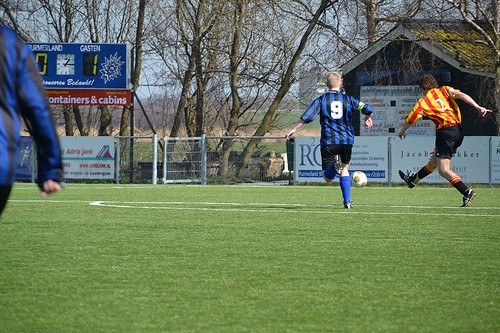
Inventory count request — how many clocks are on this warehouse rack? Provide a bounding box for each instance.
[56,52,75,76]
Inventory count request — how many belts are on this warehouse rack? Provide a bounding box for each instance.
[440,126,458,131]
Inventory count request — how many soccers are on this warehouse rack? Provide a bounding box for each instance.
[351,170,368,187]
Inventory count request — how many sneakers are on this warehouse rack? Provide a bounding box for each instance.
[345,203,353,209]
[399,170,417,188]
[460,189,476,207]
[334,155,342,175]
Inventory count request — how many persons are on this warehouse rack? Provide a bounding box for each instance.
[0,22,63,217]
[399,73,492,207]
[285,72,374,208]
